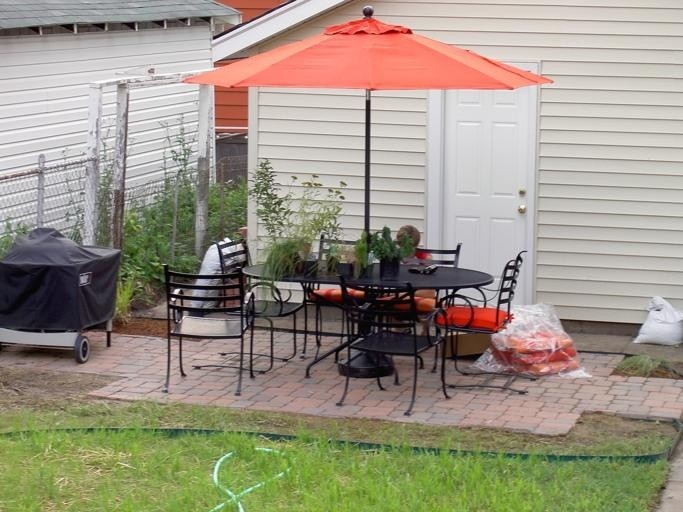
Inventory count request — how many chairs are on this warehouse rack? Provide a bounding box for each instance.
[338,277,450,415]
[163,264,254,394]
[306,232,365,347]
[191,240,304,373]
[433,250,538,394]
[373,243,462,373]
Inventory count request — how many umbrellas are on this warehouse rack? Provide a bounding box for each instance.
[181,6,554,378]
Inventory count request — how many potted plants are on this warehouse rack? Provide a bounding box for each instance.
[249,157,417,308]
